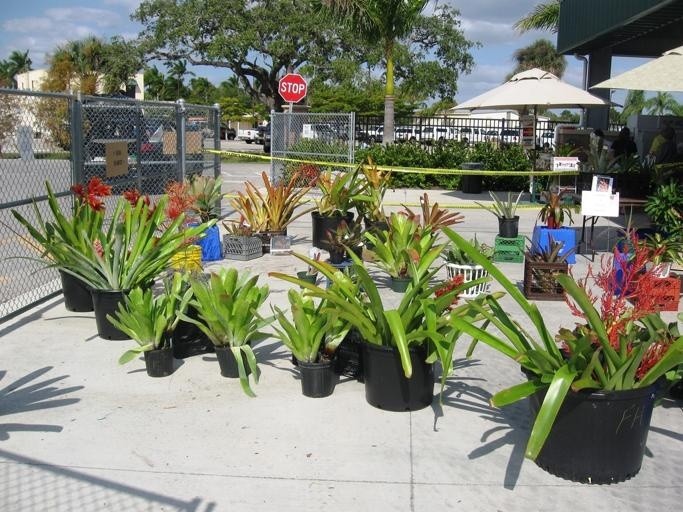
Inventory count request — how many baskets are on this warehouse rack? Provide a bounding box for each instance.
[493,235,525,263]
[445,261,488,299]
[222,234,263,261]
[523,253,568,300]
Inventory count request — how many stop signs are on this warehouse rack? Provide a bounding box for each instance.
[278,73,308,102]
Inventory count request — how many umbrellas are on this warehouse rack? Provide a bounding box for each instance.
[448,67,624,203]
[588,46,683,91]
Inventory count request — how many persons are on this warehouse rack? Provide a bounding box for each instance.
[590,129,613,148]
[649,135,667,155]
[607,126,638,170]
[655,127,678,164]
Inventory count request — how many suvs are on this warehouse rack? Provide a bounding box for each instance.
[194,121,237,140]
[77,94,205,194]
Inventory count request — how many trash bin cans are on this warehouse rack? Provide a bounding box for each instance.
[461,163,483,194]
